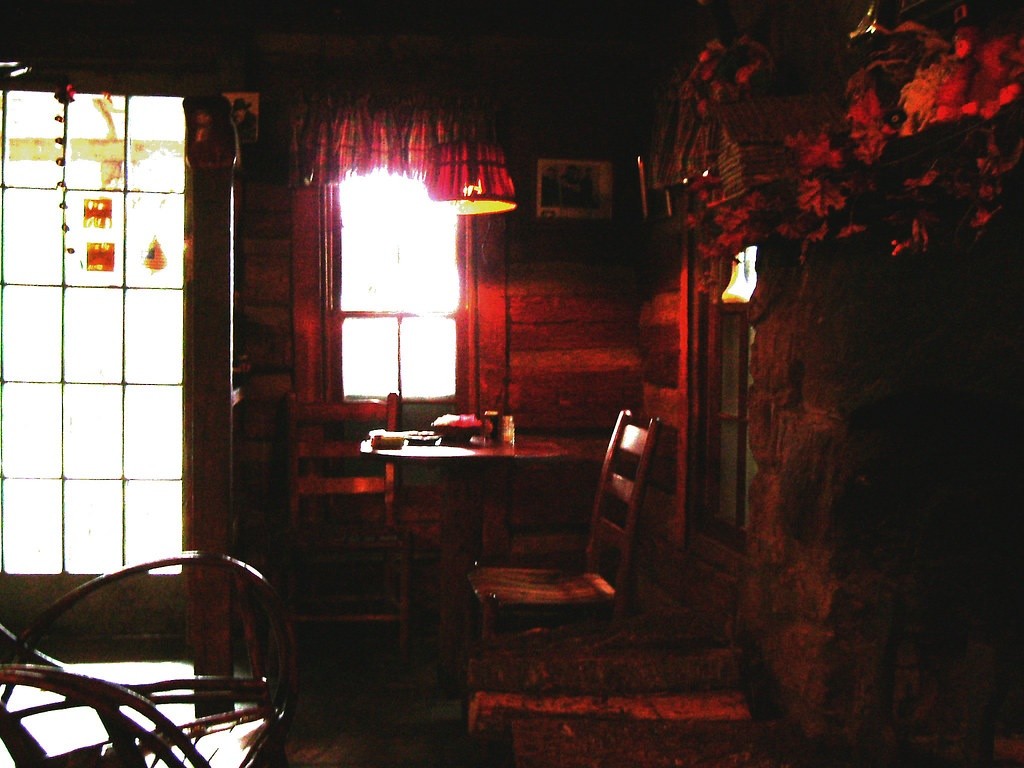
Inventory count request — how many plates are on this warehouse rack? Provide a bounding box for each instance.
[406,436,440,443]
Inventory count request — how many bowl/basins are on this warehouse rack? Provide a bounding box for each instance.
[430,424,481,443]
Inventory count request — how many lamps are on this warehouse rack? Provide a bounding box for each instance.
[416,136,519,216]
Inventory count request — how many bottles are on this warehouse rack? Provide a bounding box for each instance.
[502,415,515,446]
[386,393,400,431]
[483,411,499,443]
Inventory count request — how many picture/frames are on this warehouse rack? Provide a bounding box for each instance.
[527,153,619,222]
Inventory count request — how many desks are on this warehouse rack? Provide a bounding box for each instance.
[360,436,593,637]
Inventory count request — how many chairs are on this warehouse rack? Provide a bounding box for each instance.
[464,407,662,656]
[0,552,298,768]
[269,392,410,670]
